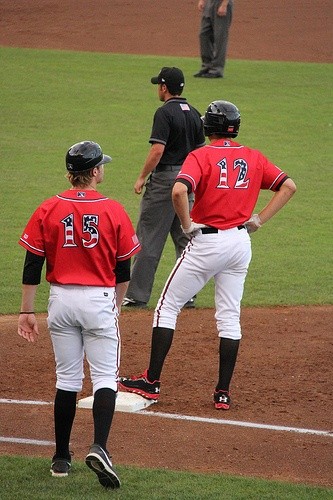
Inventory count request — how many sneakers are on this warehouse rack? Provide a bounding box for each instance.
[121,298,146,307]
[50,451,74,477]
[212,388,231,410]
[184,299,195,308]
[85,444,121,489]
[117,368,161,400]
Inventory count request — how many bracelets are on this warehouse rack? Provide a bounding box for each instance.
[18,311,35,314]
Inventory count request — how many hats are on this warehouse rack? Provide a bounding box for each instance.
[151,67,185,88]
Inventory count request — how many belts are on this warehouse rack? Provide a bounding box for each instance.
[157,165,181,171]
[200,224,245,234]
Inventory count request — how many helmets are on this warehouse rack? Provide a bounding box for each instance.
[66,141,112,172]
[200,100,241,138]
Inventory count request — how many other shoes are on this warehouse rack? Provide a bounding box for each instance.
[193,71,223,78]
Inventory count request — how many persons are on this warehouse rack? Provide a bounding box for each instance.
[119,66,205,308]
[193,0,234,78]
[14,142,141,488]
[120,101,298,408]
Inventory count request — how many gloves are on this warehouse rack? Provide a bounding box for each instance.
[245,214,262,233]
[180,222,206,240]
